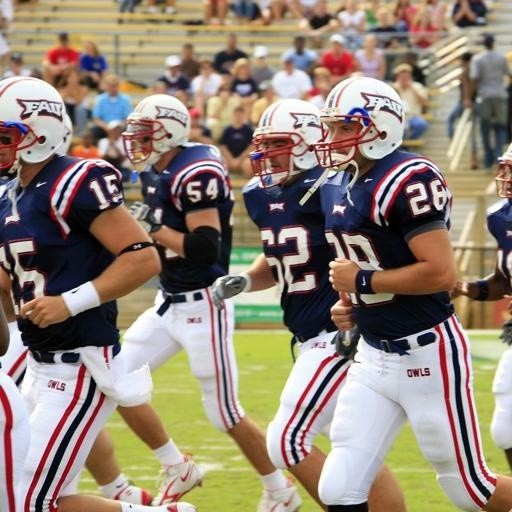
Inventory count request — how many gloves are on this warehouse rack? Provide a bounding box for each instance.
[128,199,162,237]
[210,272,248,310]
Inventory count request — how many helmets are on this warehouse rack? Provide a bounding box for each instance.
[252,98,330,170]
[322,77,405,161]
[127,93,191,154]
[1,75,69,165]
[496,139,512,170]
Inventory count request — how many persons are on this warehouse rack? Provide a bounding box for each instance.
[0,77,198,512]
[308,79,512,511]
[211,97,409,512]
[448,144,512,473]
[0,295,28,512]
[0,113,153,505]
[1,1,512,186]
[111,94,304,512]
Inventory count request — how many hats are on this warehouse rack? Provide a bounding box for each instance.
[253,43,270,59]
[164,55,184,68]
[330,34,345,45]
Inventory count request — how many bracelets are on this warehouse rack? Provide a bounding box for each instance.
[355,266,374,294]
[60,280,101,318]
[476,278,490,303]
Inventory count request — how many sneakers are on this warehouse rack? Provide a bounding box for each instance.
[150,459,204,506]
[254,484,305,512]
[111,483,154,505]
[166,501,197,512]
